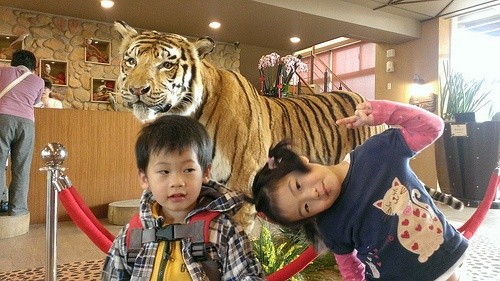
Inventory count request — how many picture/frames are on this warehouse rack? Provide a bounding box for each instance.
[84,38,111,66]
[38,59,69,87]
[0,34,24,62]
[90,77,118,104]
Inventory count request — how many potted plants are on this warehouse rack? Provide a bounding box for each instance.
[440,60,491,124]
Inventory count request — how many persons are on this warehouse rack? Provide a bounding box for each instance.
[101,114,265,281]
[37,78,62,108]
[245,100,470,281]
[0,49,44,217]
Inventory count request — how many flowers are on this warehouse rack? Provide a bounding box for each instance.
[257,52,309,98]
[247,226,314,281]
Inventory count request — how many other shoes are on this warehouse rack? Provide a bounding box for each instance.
[0,200,9,212]
